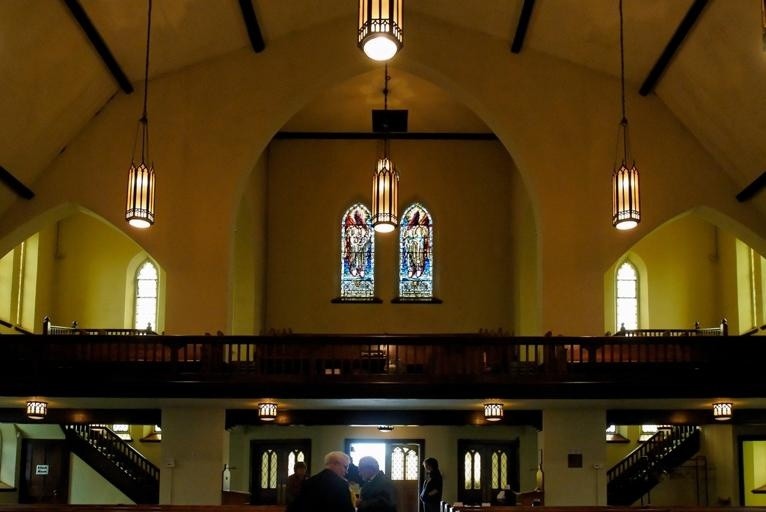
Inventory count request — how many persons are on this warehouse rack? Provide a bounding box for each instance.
[285,461,314,512]
[418,457,443,511]
[356,455,398,512]
[286,451,357,511]
[345,456,361,482]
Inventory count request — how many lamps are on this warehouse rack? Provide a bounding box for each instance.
[610,0,643,231]
[711,400,734,423]
[25,400,49,421]
[370,65,399,235]
[377,424,395,433]
[483,402,506,422]
[356,0,405,64]
[125,0,157,230]
[256,401,278,421]
